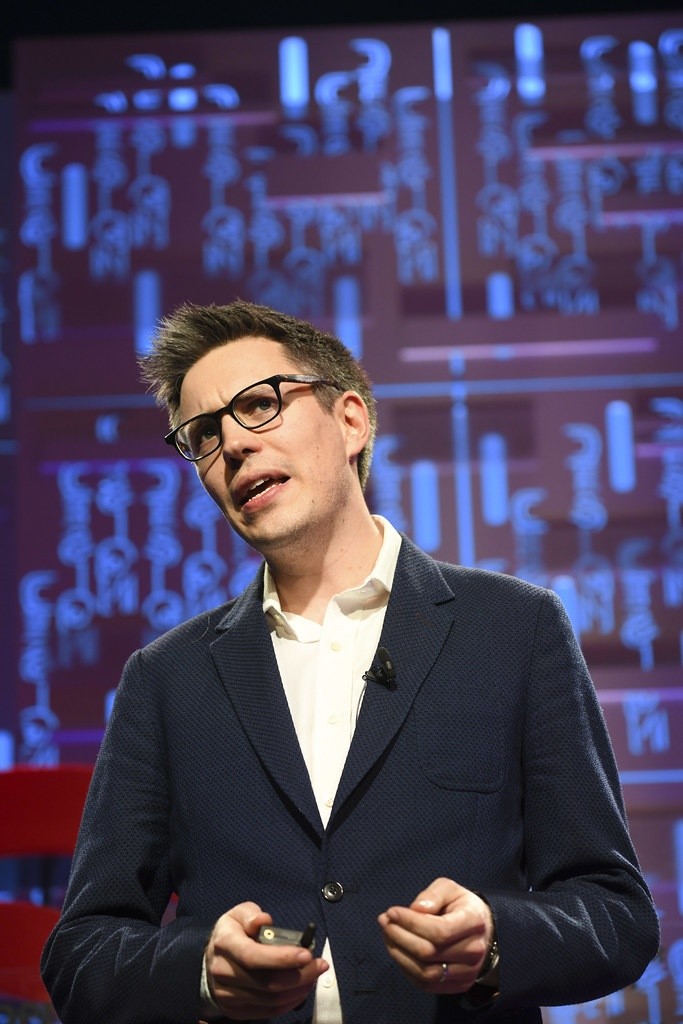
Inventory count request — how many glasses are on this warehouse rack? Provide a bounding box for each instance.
[163,373,346,462]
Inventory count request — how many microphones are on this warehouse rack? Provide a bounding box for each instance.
[362,646,398,689]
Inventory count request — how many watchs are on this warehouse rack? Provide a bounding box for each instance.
[479,917,503,982]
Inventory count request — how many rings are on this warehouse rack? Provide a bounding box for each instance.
[436,960,449,982]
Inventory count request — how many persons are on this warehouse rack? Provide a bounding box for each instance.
[38,305,663,1024]
